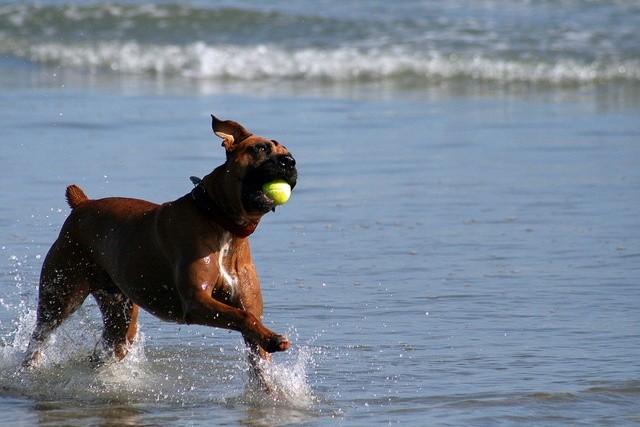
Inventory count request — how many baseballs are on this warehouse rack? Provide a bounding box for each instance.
[262,178,291,205]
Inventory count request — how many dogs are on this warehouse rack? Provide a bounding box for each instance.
[22,114,297,395]
